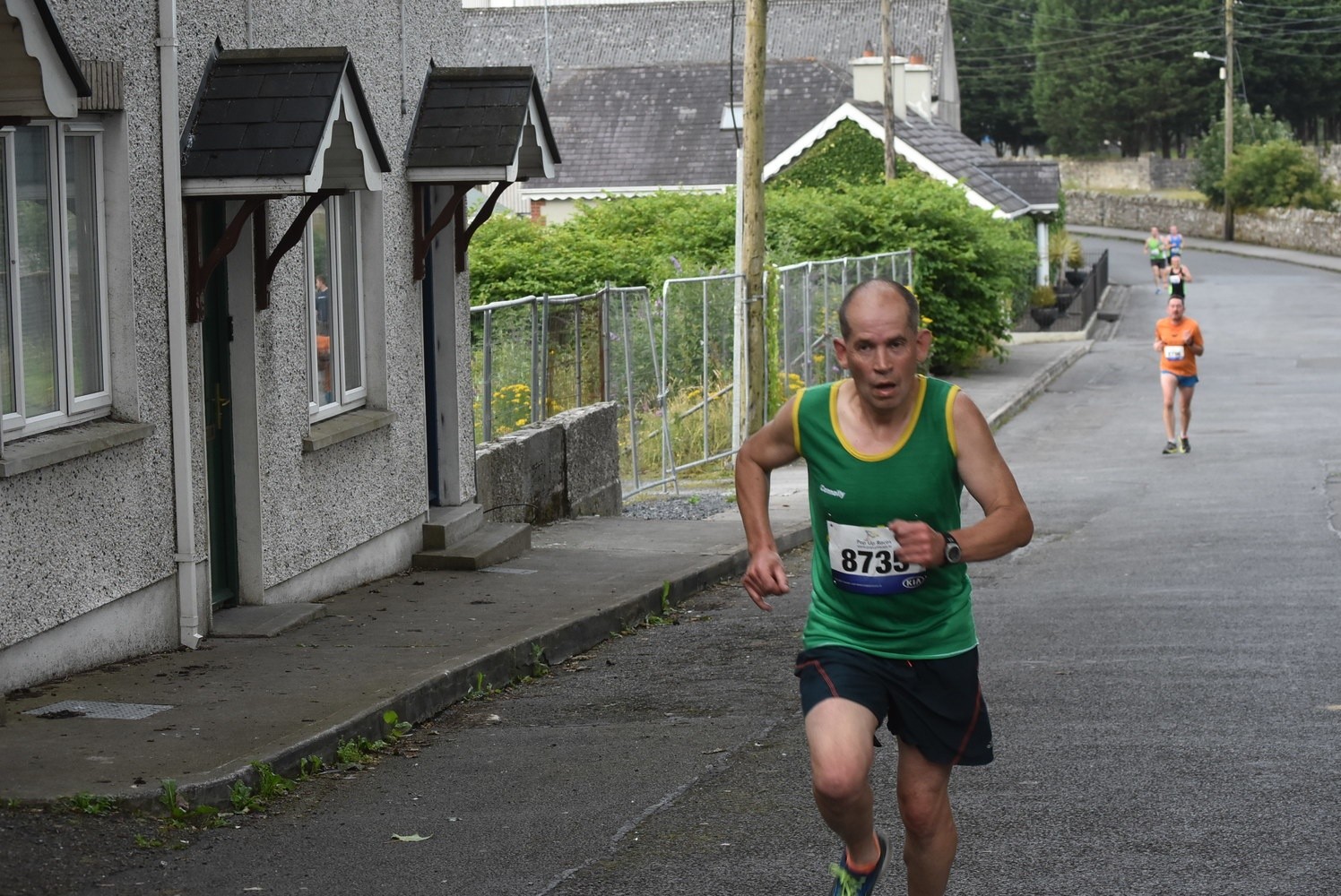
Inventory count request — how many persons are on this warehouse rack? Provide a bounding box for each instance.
[310,273,335,406]
[1144,226,1168,295]
[1166,226,1185,264]
[734,276,1034,896]
[1153,294,1204,454]
[1162,253,1192,300]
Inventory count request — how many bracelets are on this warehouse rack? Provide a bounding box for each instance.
[1184,341,1194,347]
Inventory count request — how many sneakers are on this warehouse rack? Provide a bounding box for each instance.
[831,832,892,896]
[1178,435,1190,453]
[1163,442,1175,453]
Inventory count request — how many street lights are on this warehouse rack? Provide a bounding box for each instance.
[1193,51,1235,242]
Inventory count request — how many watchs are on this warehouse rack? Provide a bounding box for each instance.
[939,530,962,569]
